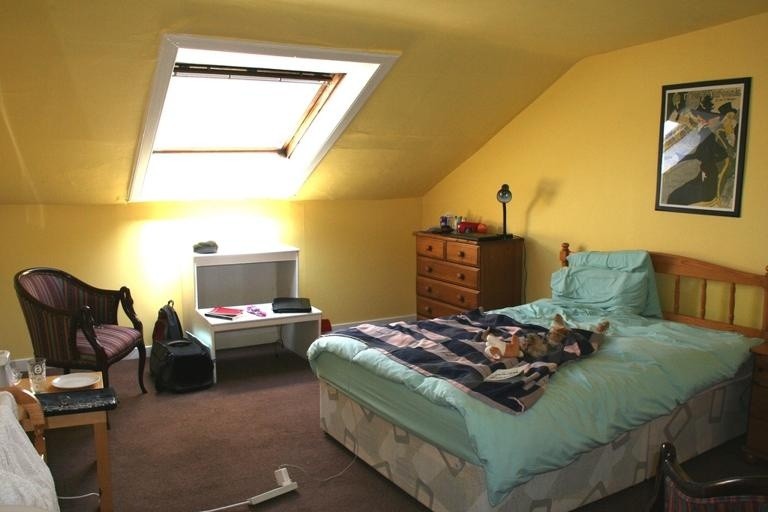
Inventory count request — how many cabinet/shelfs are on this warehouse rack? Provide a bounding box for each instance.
[412,229,524,322]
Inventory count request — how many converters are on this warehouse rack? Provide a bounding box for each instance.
[274,468,291,486]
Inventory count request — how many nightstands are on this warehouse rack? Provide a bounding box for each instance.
[745,340,768,462]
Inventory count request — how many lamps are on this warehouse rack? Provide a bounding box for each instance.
[495,183,513,240]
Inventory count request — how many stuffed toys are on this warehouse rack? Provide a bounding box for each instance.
[484,334,524,363]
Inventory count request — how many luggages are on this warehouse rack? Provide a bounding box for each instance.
[149,338,214,395]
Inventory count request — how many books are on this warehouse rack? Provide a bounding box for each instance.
[205,305,244,320]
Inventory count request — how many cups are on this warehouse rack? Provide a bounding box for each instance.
[25,357,47,394]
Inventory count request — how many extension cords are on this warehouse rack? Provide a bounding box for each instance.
[246,480,299,506]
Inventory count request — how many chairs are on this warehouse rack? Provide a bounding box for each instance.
[14,266,148,400]
[640,440,768,512]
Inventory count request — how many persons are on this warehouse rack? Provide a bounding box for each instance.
[665,103,738,207]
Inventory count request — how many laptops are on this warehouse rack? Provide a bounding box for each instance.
[457,233,500,241]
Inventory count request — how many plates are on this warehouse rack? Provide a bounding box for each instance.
[51,373,100,389]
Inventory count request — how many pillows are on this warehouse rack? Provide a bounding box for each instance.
[551,266,648,318]
[565,251,664,320]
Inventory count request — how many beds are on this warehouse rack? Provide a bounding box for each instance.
[306,242,768,511]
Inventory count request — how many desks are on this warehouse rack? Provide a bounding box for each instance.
[13,373,112,511]
[197,298,321,386]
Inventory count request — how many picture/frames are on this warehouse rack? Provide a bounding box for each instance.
[654,76,753,219]
